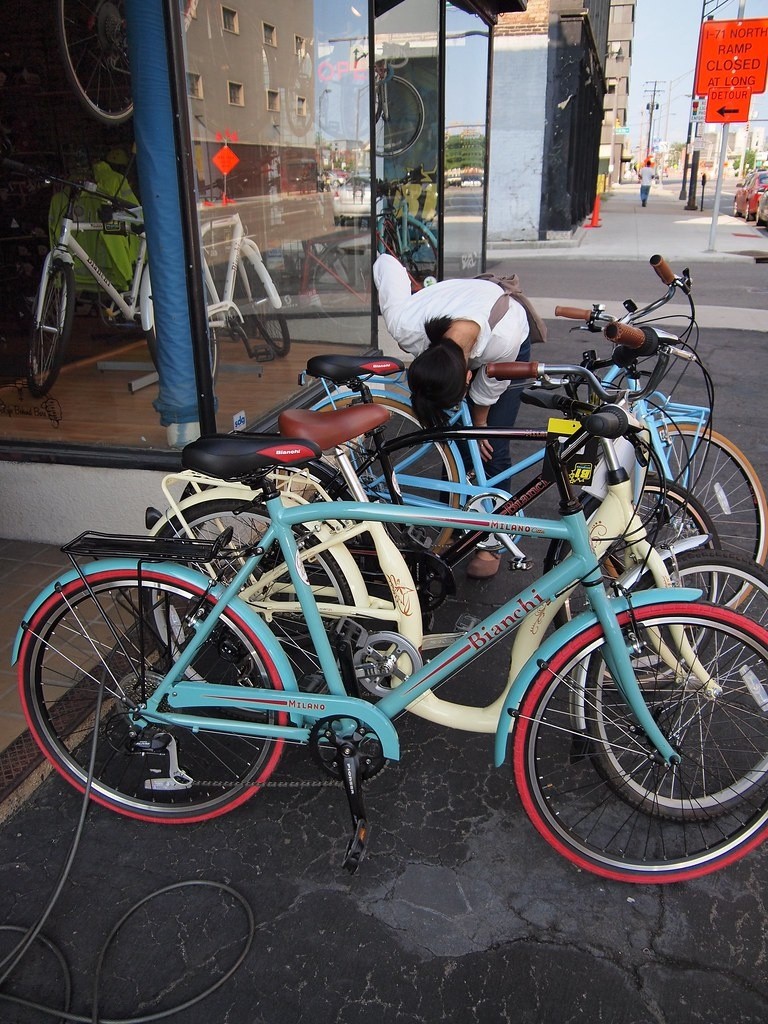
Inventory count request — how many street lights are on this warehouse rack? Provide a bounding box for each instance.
[318,88,333,191]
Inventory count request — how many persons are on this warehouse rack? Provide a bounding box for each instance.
[373,253,530,575]
[48,157,148,293]
[638,161,654,207]
[317,172,330,192]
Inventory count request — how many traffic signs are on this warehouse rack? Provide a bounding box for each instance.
[705,85,752,123]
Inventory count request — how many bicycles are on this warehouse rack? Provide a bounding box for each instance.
[1,153,768,887]
[53,0,133,128]
[375,41,426,159]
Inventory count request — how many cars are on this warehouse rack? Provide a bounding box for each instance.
[755,186,768,227]
[733,170,768,221]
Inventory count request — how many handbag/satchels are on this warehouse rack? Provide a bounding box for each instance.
[481,273,548,344]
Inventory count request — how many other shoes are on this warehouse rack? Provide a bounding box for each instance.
[467,550,502,579]
[441,541,454,554]
